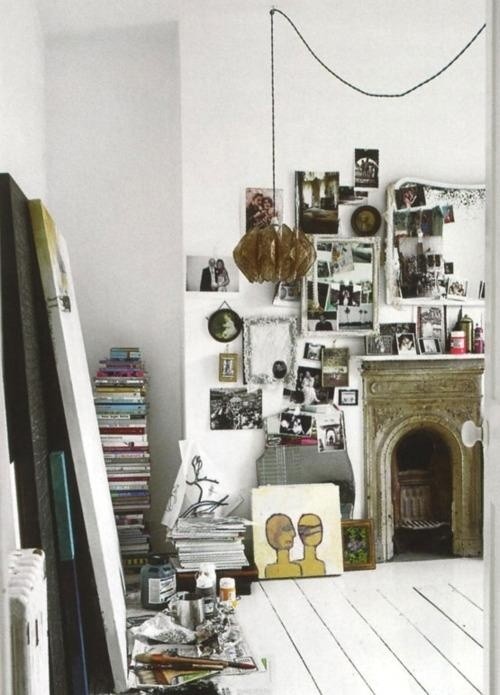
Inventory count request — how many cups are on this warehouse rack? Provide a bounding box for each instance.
[170,592,204,630]
[450,331,466,352]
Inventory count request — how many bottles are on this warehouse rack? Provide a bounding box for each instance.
[219,577,237,610]
[459,314,473,353]
[195,562,217,619]
[474,323,484,353]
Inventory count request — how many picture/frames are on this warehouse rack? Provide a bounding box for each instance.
[339,518,377,572]
[218,353,239,382]
[338,388,359,407]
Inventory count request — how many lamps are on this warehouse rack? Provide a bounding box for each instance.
[232,8,486,288]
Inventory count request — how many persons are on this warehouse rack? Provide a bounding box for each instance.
[407,213,432,237]
[401,188,418,208]
[316,314,333,330]
[198,259,230,291]
[399,335,416,354]
[334,288,357,308]
[283,415,304,434]
[296,371,330,403]
[449,281,465,296]
[246,192,277,232]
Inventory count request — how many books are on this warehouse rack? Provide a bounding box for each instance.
[91,358,262,569]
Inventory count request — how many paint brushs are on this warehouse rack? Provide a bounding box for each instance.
[135,653,256,669]
